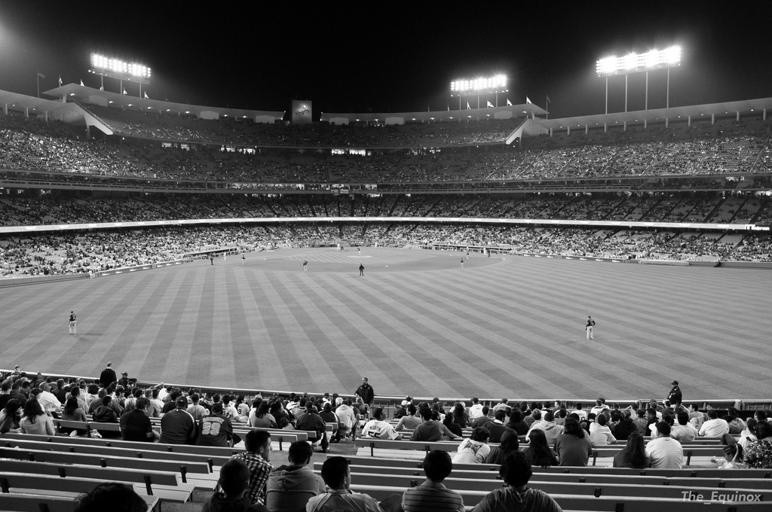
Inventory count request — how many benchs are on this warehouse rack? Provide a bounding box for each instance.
[0,371,770,510]
[211,118,461,251]
[461,114,772,267]
[0,99,210,283]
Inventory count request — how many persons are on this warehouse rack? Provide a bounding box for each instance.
[0,362,770,470]
[471,451,568,511]
[583,315,595,341]
[227,430,273,504]
[305,456,382,511]
[402,449,464,511]
[266,441,324,512]
[200,460,272,511]
[1,104,771,279]
[72,481,148,512]
[67,309,79,337]
